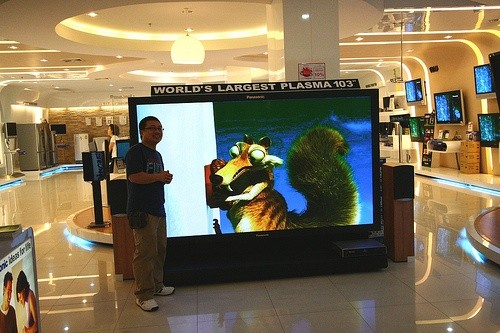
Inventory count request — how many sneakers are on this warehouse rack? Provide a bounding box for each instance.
[153,287,175,295]
[136,298,159,311]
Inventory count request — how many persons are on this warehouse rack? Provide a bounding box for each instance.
[125,116,175,312]
[108,124,120,172]
[0,272,18,333]
[16,270,38,333]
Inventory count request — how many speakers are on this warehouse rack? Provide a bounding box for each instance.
[82,152,105,181]
[108,175,135,280]
[382,164,415,263]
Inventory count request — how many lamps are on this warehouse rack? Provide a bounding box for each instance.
[170,32,205,65]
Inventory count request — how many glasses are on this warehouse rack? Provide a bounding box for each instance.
[142,127,164,131]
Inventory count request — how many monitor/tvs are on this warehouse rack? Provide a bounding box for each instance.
[474,63,495,94]
[390,114,410,128]
[434,89,466,126]
[116,140,130,159]
[478,112,500,148]
[405,78,423,103]
